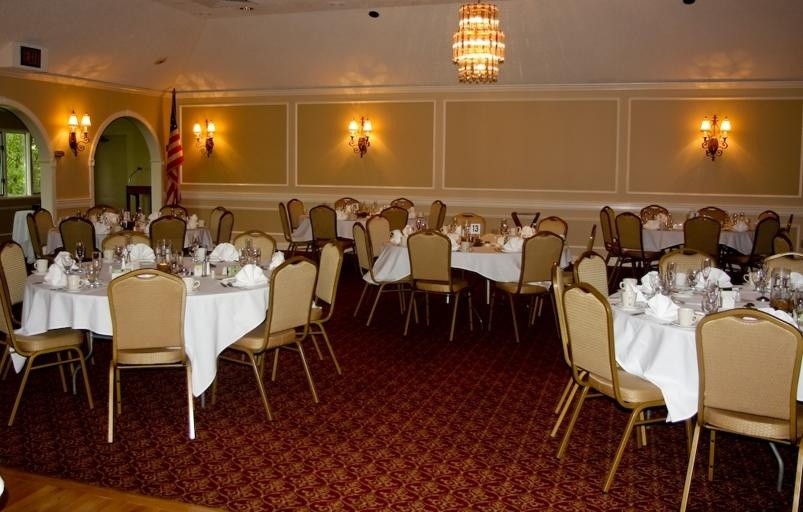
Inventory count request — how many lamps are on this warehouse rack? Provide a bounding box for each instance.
[67,109,92,157]
[348,117,370,158]
[192,120,214,158]
[451,0,505,83]
[700,115,730,161]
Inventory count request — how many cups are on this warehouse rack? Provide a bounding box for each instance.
[393,211,538,253]
[29,236,261,295]
[608,258,803,336]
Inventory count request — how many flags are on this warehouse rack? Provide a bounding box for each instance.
[164,93,185,207]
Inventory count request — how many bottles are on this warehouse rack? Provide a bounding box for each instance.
[301,198,391,221]
[56,205,206,235]
[641,208,759,232]
